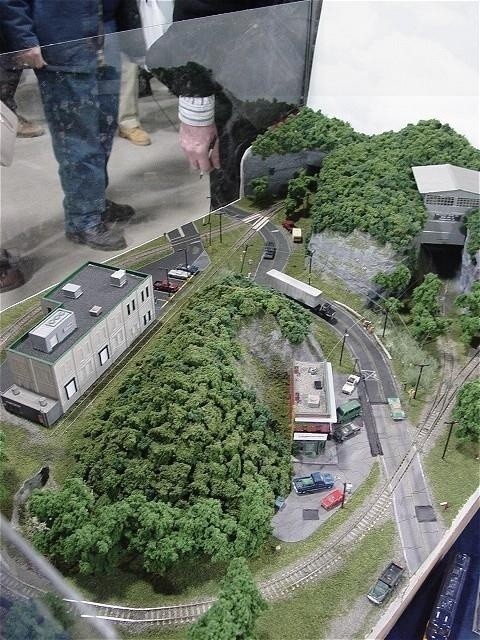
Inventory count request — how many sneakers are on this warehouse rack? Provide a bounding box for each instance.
[64,220,128,251]
[99,197,134,221]
[118,122,152,146]
[9,110,46,138]
[0,252,25,293]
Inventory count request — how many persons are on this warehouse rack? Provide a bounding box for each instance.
[2,1,325,293]
